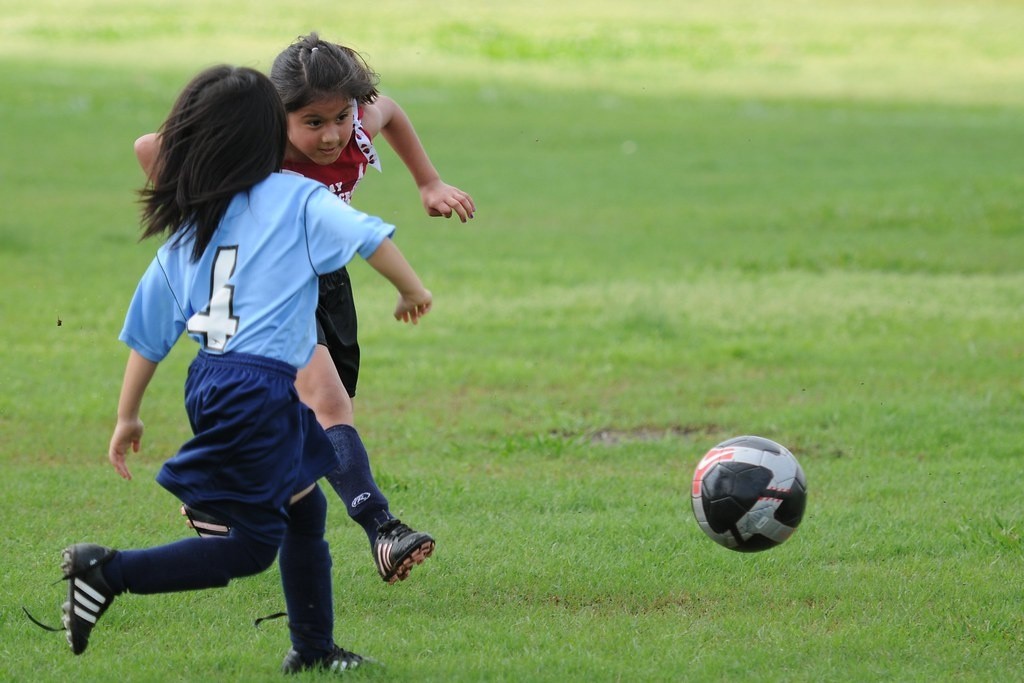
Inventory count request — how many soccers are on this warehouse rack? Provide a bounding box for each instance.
[690,435,808,553]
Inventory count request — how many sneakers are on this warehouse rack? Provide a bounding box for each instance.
[280,646,387,673]
[179,502,229,539]
[20,544,119,656]
[371,517,437,587]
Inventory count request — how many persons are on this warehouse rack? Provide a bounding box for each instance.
[60,65,432,673]
[134,31,475,583]
